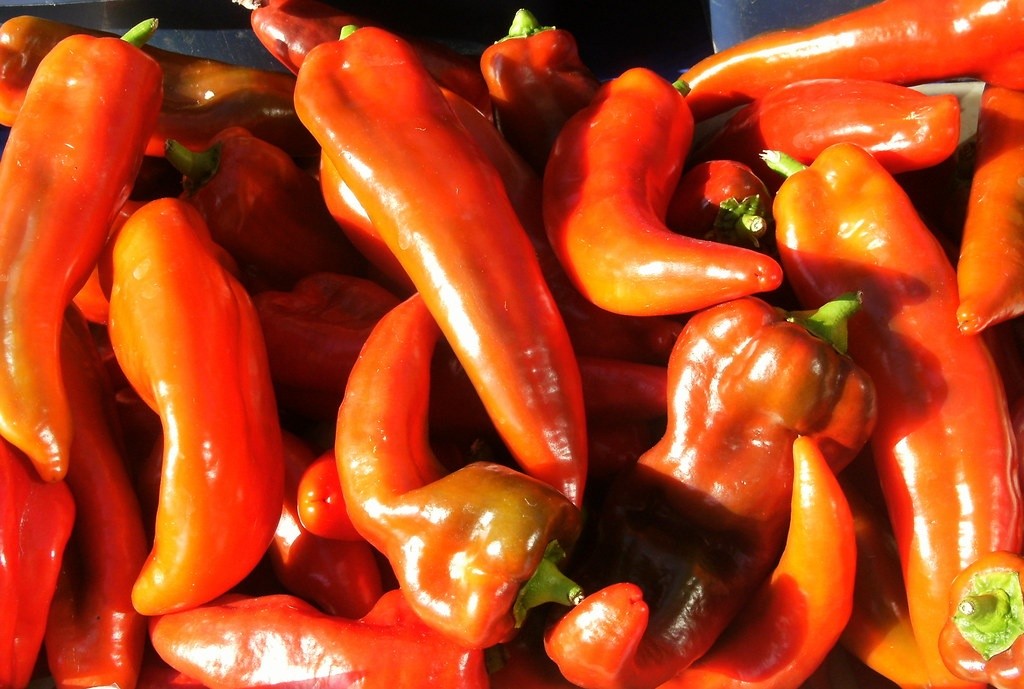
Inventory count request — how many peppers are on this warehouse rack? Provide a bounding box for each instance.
[0,0,1024,689]
